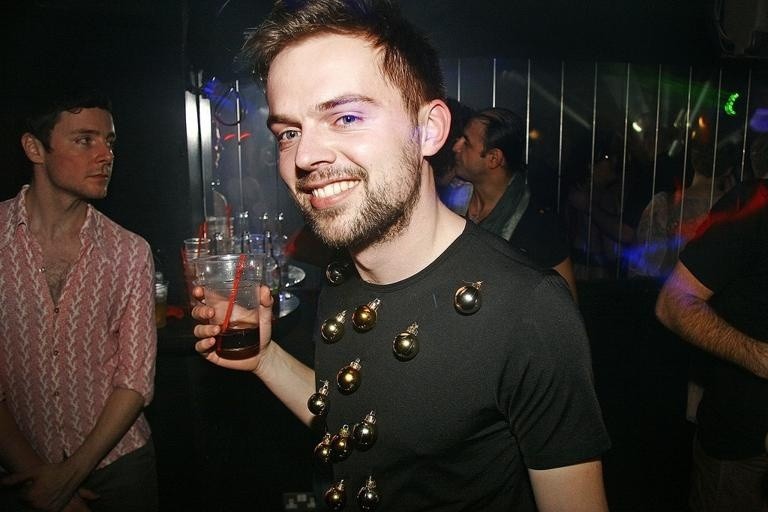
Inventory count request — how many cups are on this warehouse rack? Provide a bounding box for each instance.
[155,281,169,328]
[180,219,295,360]
[155,271,163,284]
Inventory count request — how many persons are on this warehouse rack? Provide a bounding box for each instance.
[565,133,738,429]
[188,0,612,511]
[0,86,165,510]
[453,96,575,300]
[431,97,469,215]
[653,124,765,510]
